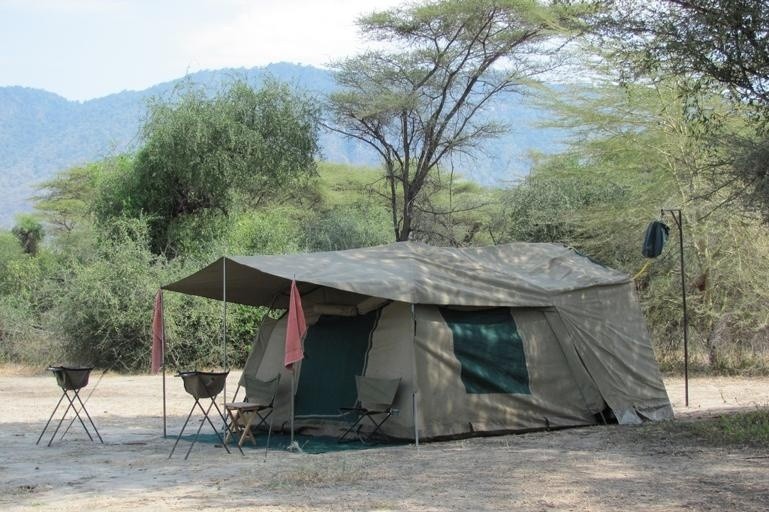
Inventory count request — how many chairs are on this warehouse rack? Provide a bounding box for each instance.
[337,371,404,447]
[223,375,280,448]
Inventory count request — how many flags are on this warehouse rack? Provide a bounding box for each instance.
[149,290,166,374]
[281,280,308,373]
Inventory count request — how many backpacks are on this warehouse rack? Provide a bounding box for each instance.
[642,220,670,258]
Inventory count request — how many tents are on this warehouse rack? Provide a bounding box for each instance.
[152,238,676,453]
